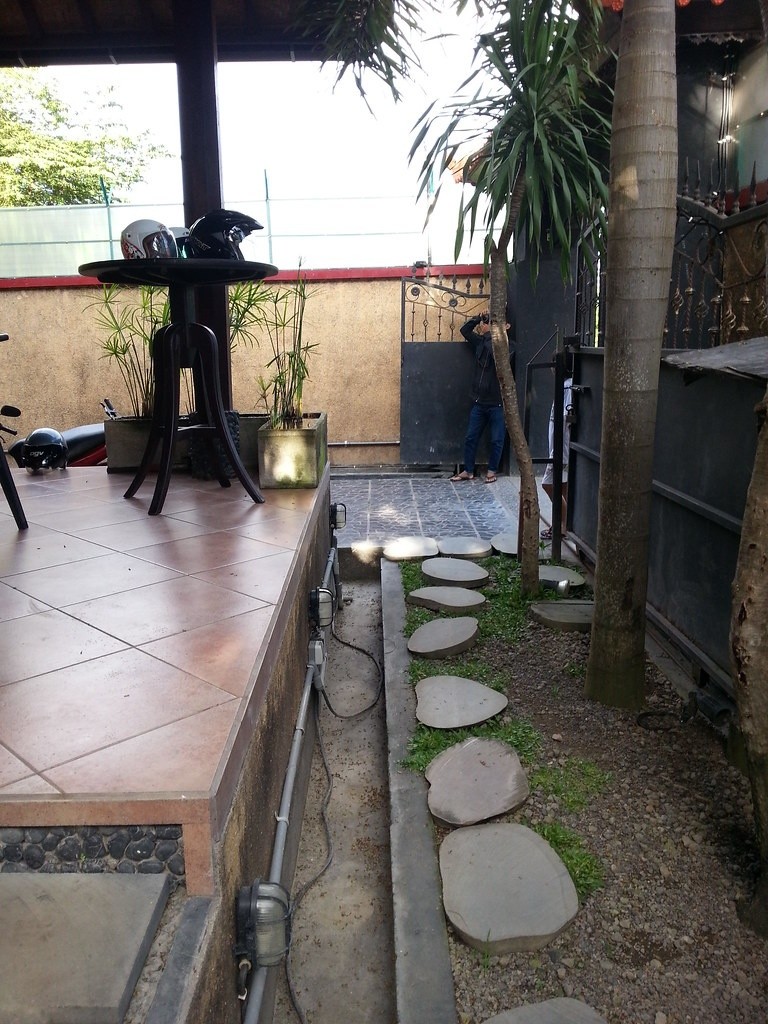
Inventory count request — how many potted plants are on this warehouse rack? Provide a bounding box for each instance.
[82,258,328,489]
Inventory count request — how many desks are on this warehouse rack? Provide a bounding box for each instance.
[79,257,279,515]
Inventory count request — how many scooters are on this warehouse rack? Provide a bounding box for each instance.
[0,334,107,467]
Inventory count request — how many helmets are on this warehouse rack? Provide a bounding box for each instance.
[184,209,264,260]
[157,226,191,258]
[120,218,179,259]
[21,427,70,472]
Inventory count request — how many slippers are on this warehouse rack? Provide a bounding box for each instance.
[449,473,474,481]
[485,475,497,484]
[540,526,567,540]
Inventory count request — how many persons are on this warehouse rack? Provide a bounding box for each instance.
[449,309,517,483]
[540,349,574,540]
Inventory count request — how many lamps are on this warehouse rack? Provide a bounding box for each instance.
[330,503,347,530]
[310,587,334,630]
